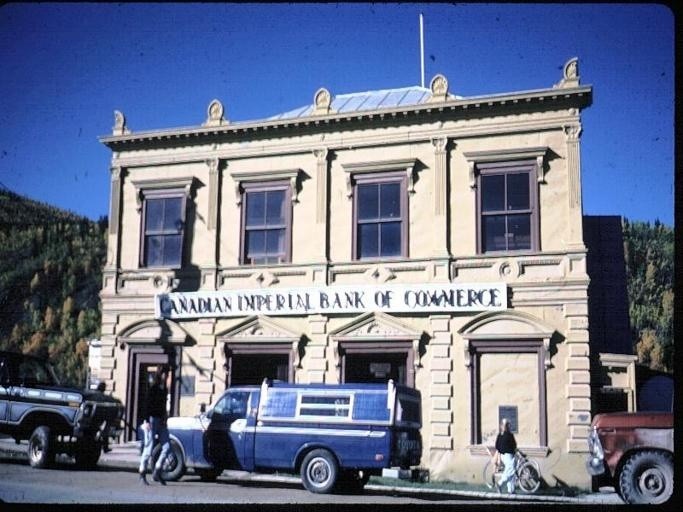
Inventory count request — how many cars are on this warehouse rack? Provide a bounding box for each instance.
[586,410,675,505]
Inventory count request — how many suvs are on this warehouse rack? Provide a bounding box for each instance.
[0,350,125,469]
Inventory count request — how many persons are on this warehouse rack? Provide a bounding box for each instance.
[95,382,112,453]
[494,418,517,494]
[138,363,172,486]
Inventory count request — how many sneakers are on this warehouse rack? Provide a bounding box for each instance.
[136,472,167,488]
[492,486,517,496]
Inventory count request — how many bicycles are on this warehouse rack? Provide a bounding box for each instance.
[483,447,541,493]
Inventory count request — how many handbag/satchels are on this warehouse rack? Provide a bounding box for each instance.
[489,449,503,467]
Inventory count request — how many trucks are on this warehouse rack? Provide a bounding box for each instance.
[138,377,422,494]
